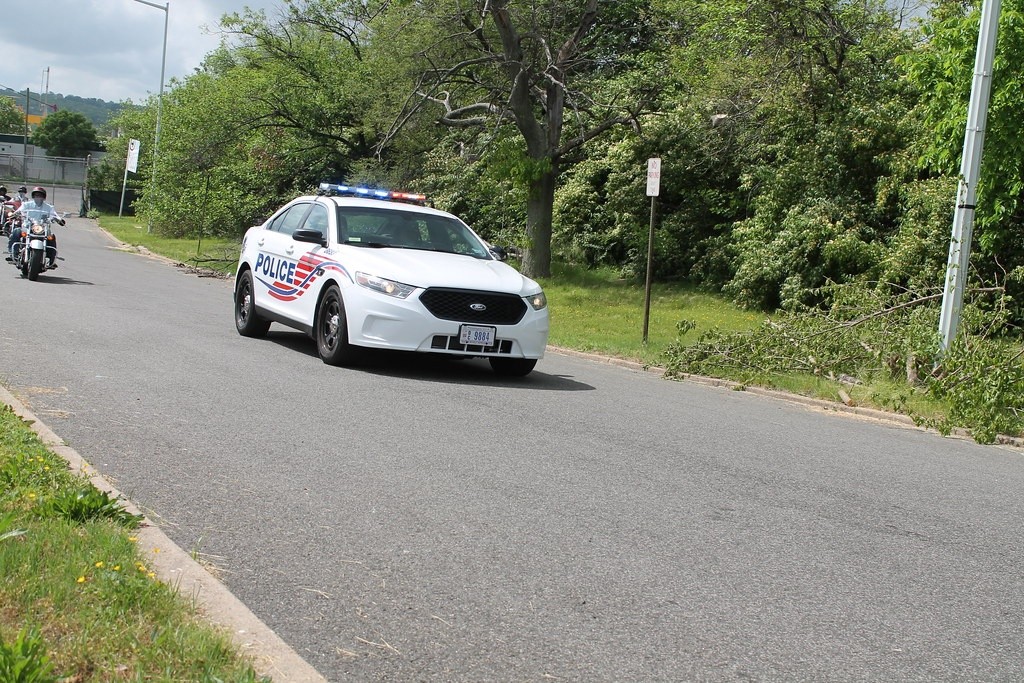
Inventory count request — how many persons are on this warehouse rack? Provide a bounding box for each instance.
[5,186,65,268]
[0,186,12,203]
[0,186,30,233]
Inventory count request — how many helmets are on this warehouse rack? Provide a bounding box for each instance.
[0,186,7,193]
[32,187,46,199]
[18,187,27,193]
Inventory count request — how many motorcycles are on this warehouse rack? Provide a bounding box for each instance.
[0,196,30,240]
[1,197,71,281]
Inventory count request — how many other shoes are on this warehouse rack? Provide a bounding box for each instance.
[47,261,58,268]
[5,257,18,262]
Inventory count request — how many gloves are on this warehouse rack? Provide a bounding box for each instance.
[58,219,65,226]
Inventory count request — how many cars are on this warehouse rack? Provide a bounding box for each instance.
[234,182,550,382]
